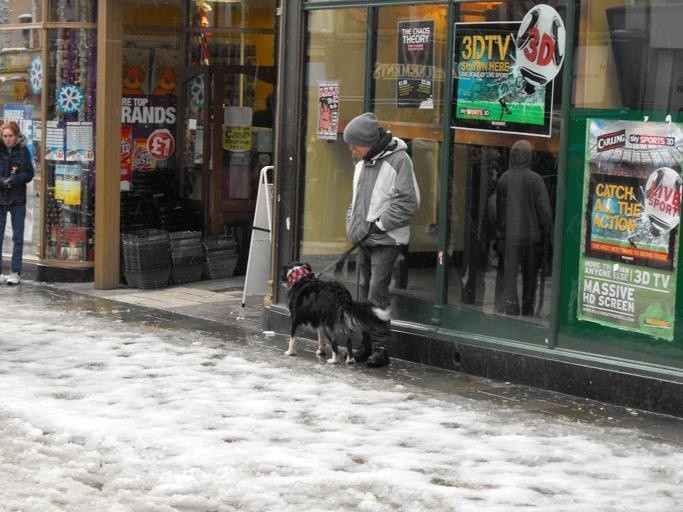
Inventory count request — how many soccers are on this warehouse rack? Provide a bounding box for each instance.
[644,167,681,236]
[515,3,566,85]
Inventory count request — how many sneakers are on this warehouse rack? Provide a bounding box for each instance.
[346,347,370,362]
[7,272,20,284]
[367,350,389,367]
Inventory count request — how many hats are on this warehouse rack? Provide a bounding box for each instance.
[509,139,530,164]
[343,112,381,146]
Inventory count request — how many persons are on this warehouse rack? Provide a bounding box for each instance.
[341,111,423,368]
[0,119,36,285]
[494,139,554,316]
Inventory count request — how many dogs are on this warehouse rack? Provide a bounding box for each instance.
[281,261,392,365]
